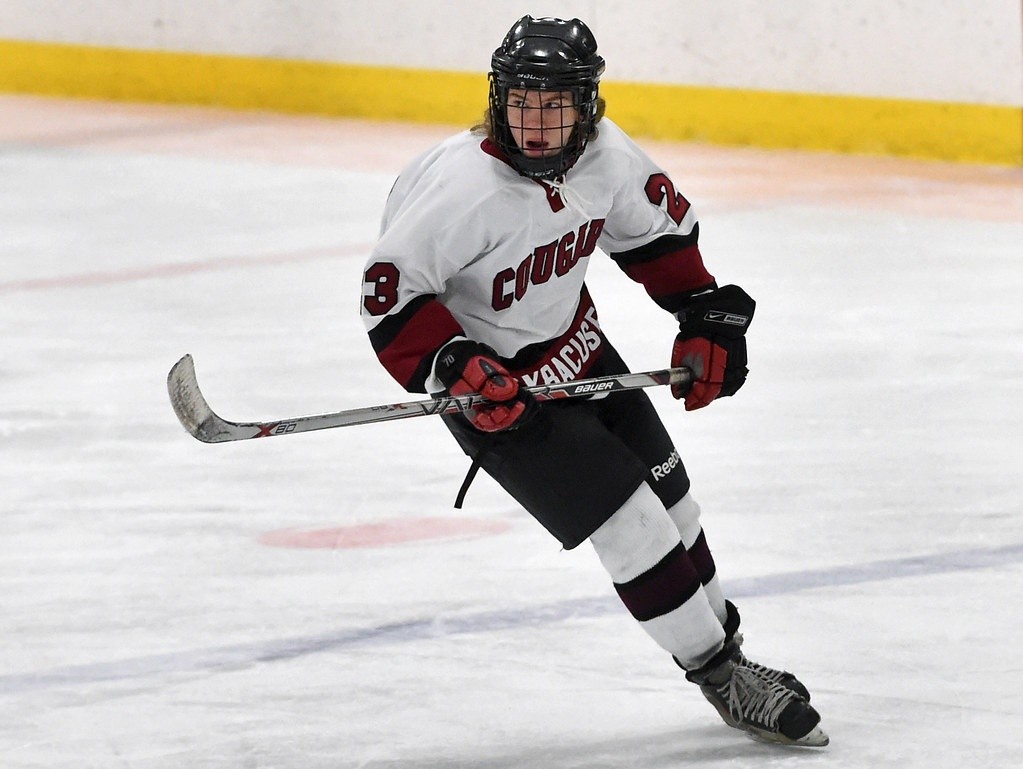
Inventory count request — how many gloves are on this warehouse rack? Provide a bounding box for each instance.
[670,284,756,411]
[435,340,543,433]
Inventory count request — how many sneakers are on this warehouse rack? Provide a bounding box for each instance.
[723,599,811,703]
[672,639,830,747]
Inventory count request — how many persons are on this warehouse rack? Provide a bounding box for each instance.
[359,14,829,747]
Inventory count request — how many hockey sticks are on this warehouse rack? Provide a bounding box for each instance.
[163,349,693,446]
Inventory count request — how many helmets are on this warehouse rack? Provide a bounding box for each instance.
[487,14,606,178]
